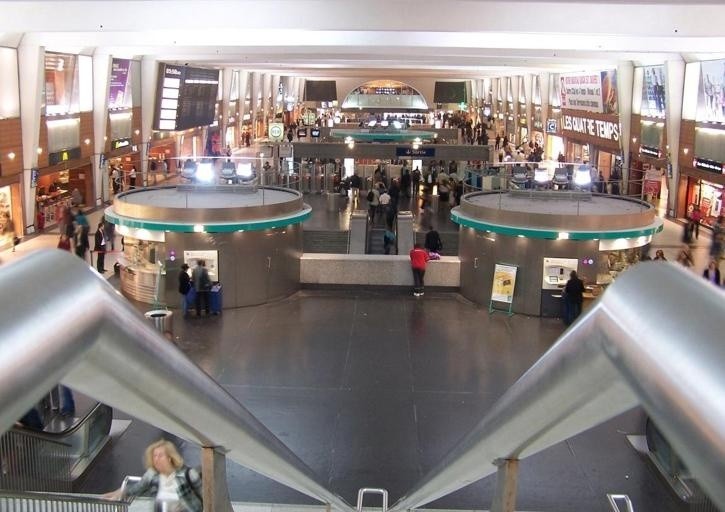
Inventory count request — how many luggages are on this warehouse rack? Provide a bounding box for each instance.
[210,284,222,315]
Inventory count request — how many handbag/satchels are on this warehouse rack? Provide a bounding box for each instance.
[438,243,442,250]
[187,288,195,303]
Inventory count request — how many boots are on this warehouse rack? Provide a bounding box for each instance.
[414,288,424,296]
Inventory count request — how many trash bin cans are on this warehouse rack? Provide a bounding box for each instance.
[144,310,172,342]
[326,193,341,213]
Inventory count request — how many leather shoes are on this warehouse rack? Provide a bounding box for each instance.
[99,269,106,273]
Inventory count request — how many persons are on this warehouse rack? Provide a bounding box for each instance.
[178,264,192,318]
[104,438,203,512]
[643,205,725,286]
[367,185,443,254]
[410,243,430,296]
[109,163,137,194]
[94,213,124,273]
[564,270,586,325]
[151,110,621,196]
[55,196,89,258]
[15,408,41,432]
[192,258,211,316]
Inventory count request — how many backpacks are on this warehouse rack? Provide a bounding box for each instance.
[367,191,373,201]
[429,251,440,260]
